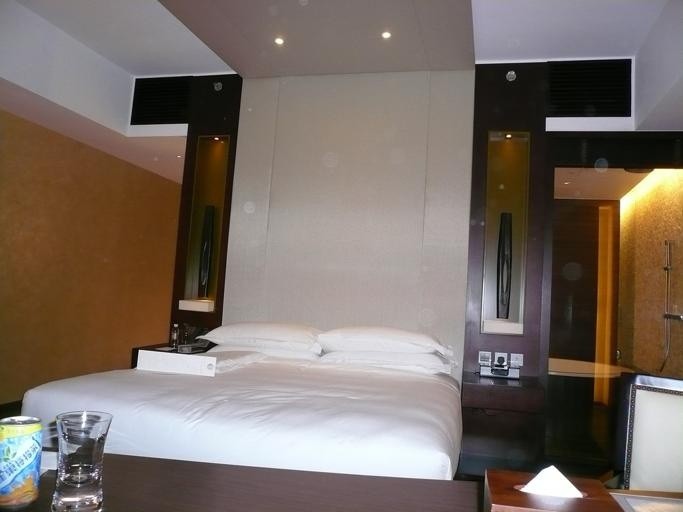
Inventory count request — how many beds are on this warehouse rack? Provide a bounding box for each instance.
[21,351,461,480]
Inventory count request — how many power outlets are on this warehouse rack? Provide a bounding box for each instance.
[494,352,508,366]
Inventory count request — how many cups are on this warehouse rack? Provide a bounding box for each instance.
[49,409,113,512]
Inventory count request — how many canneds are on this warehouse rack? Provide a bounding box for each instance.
[0,416,43,507]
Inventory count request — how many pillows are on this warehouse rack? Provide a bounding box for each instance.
[195,321,459,375]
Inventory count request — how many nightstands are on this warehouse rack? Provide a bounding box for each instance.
[462,371,545,463]
[131,343,210,370]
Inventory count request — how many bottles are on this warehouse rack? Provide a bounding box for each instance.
[170,324,179,347]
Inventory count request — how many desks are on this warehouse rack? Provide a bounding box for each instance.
[0,447,478,512]
